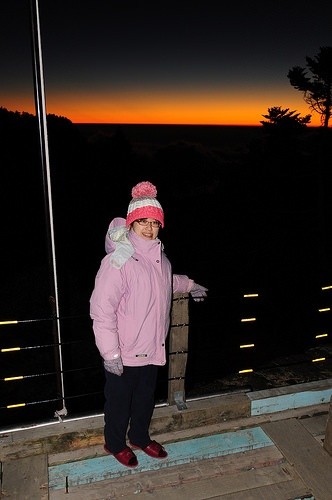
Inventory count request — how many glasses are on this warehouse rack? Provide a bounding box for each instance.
[135,219,162,227]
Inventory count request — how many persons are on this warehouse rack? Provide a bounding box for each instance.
[90,182,209,468]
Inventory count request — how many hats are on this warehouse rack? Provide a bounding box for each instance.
[126,181,164,228]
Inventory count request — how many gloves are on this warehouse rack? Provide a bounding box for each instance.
[190,282,208,302]
[104,357,123,376]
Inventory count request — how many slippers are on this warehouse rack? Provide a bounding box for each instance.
[129,439,167,458]
[104,444,139,468]
